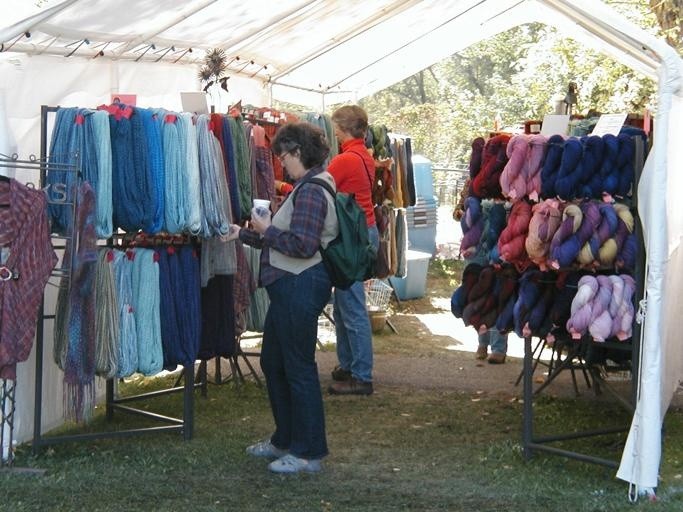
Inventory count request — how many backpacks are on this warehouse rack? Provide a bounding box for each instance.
[291,178,378,290]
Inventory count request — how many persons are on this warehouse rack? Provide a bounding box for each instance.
[269,104,383,396]
[475,325,509,364]
[216,119,341,476]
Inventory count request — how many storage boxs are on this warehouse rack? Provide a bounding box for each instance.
[390,250,432,300]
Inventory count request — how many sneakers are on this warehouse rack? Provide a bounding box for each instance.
[476,345,505,363]
[327,366,372,394]
[246,439,321,473]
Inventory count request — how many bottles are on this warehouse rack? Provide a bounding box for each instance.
[251,197,274,221]
